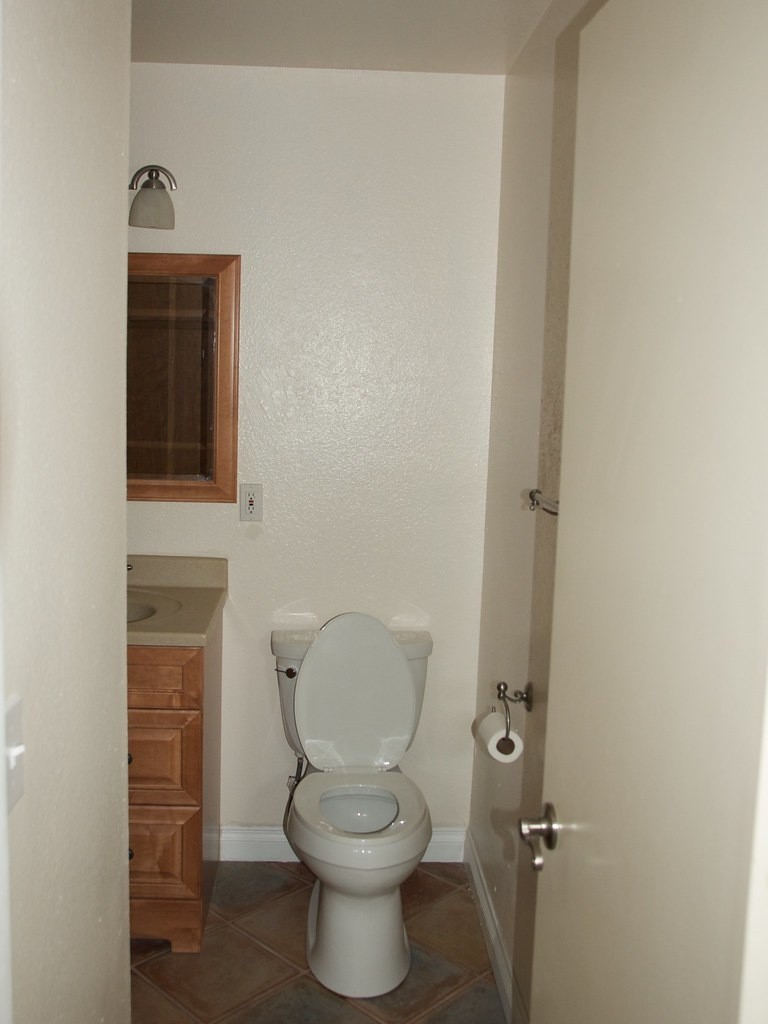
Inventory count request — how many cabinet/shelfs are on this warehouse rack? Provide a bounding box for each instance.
[128,609,223,954]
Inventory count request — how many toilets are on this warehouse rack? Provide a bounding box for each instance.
[268,609,435,1000]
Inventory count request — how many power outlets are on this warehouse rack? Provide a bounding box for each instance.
[240,484,264,521]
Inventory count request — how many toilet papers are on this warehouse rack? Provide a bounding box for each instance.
[477,711,525,764]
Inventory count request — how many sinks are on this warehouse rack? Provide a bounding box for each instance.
[127,587,184,625]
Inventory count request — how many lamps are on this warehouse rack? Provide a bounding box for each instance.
[128,165,177,231]
